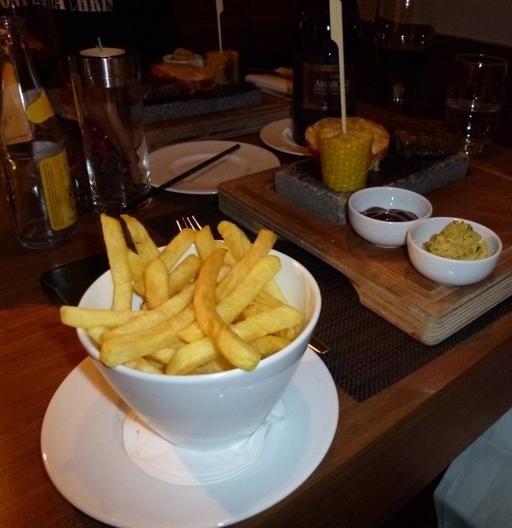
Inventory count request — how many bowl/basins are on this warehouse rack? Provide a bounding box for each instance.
[406,216,503,286]
[162,53,204,69]
[75,239,322,453]
[347,185,433,249]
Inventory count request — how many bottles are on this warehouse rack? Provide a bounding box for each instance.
[291,0,363,147]
[0,15,81,250]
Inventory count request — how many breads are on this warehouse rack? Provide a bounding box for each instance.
[305,115,390,171]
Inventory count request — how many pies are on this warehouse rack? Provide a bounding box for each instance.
[422,220,488,260]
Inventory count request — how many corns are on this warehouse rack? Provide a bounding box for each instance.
[317,125,374,193]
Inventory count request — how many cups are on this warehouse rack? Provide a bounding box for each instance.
[67,46,154,219]
[445,54,510,159]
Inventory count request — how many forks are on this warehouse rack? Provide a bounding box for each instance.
[175,215,332,355]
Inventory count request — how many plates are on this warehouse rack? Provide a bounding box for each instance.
[259,116,390,157]
[40,345,341,526]
[145,139,281,196]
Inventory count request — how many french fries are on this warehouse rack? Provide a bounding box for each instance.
[58,213,304,373]
